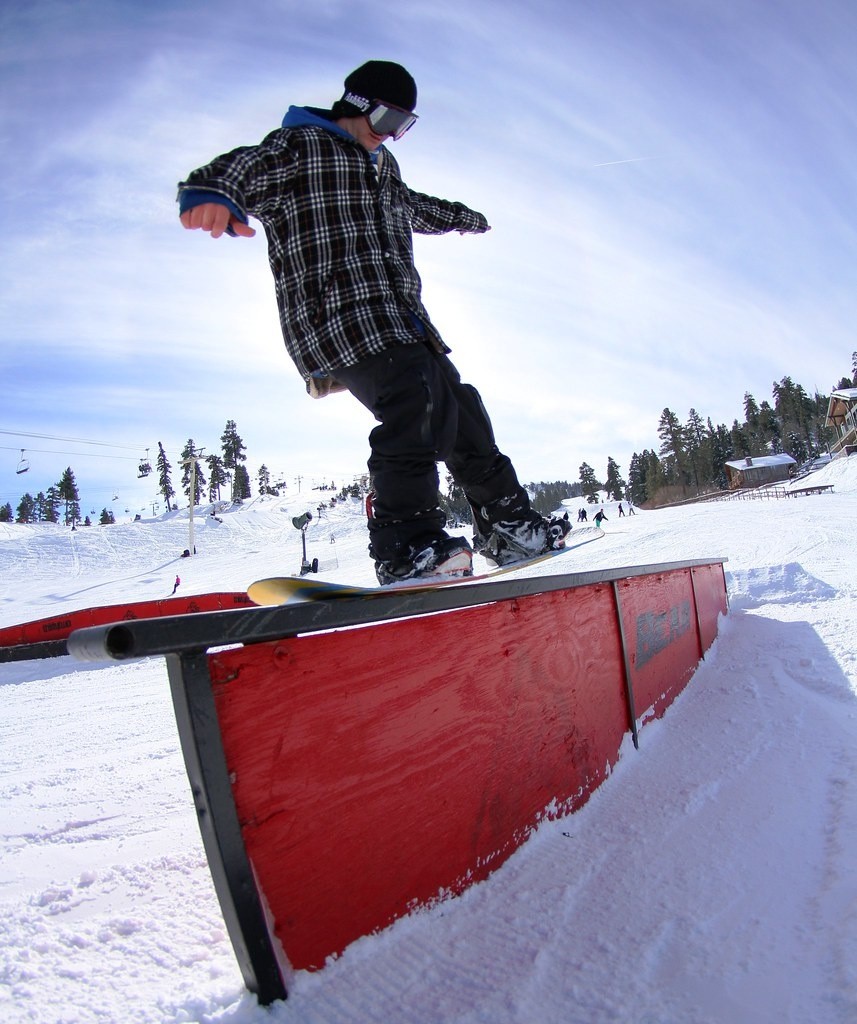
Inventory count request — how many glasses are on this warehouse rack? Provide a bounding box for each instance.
[363,101,419,142]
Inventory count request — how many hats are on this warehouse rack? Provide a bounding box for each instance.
[344,58,417,112]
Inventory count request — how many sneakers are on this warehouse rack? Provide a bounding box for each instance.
[363,507,471,587]
[474,497,571,555]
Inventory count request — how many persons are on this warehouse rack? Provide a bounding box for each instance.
[581,508,587,522]
[577,509,582,522]
[172,575,180,594]
[330,534,335,544]
[139,463,152,475]
[628,501,635,516]
[593,508,608,527]
[563,511,569,520]
[618,503,626,517]
[176,61,572,583]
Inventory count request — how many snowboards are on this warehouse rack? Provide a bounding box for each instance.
[245,525,607,606]
